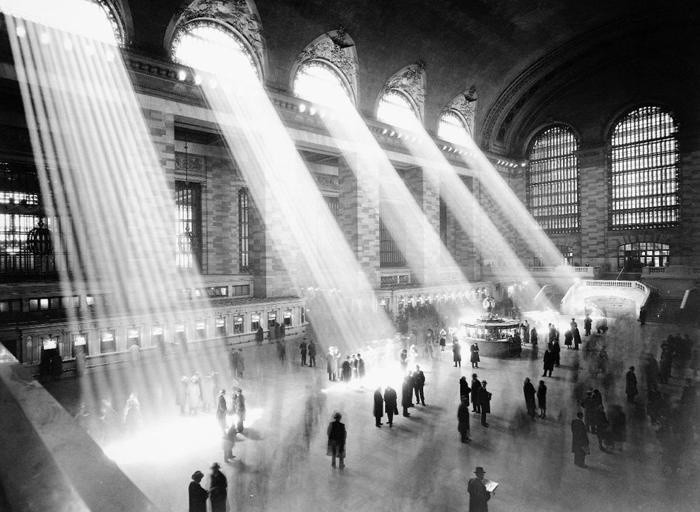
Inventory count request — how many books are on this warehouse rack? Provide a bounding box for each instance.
[485,480,499,493]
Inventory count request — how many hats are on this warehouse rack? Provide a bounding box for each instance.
[473,466,486,475]
[333,413,341,419]
[210,462,221,468]
[481,380,487,384]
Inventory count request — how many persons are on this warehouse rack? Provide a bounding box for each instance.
[640,307,646,324]
[457,374,492,442]
[508,311,609,377]
[255,319,316,368]
[325,345,365,382]
[70,393,143,437]
[608,333,700,443]
[305,376,347,469]
[402,329,480,368]
[468,466,491,512]
[571,389,610,468]
[189,463,227,512]
[176,348,247,463]
[523,378,548,419]
[374,365,427,429]
